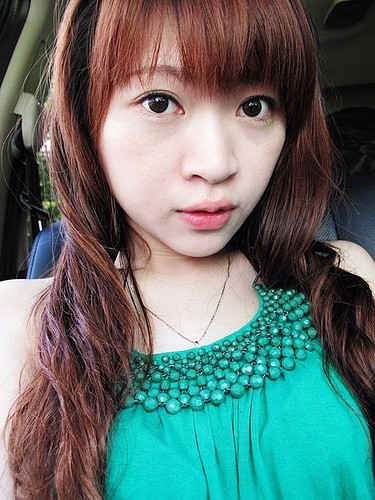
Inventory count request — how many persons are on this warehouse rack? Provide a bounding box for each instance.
[0,0,375,500]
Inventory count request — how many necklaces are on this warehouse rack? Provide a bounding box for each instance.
[126,249,231,346]
